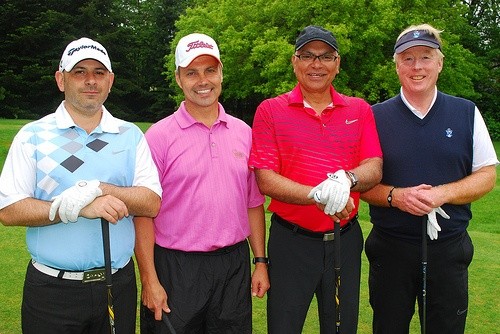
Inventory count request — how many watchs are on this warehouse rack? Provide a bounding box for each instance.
[345,169,358,190]
[388,187,395,208]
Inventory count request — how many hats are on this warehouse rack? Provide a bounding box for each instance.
[59,37,112,73]
[393,29,440,54]
[174,33,224,68]
[295,25,339,51]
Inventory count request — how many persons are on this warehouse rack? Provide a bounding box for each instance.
[359,23,500,334]
[247,26,384,334]
[0,37,163,334]
[132,31,271,334]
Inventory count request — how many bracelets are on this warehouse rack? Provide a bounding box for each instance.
[252,256,268,265]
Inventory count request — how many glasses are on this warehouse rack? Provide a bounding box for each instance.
[295,54,338,62]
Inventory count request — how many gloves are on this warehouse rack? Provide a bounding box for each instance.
[307,169,352,216]
[426,207,451,241]
[49,179,103,224]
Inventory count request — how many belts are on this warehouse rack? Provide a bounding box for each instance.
[272,212,357,241]
[31,261,129,284]
